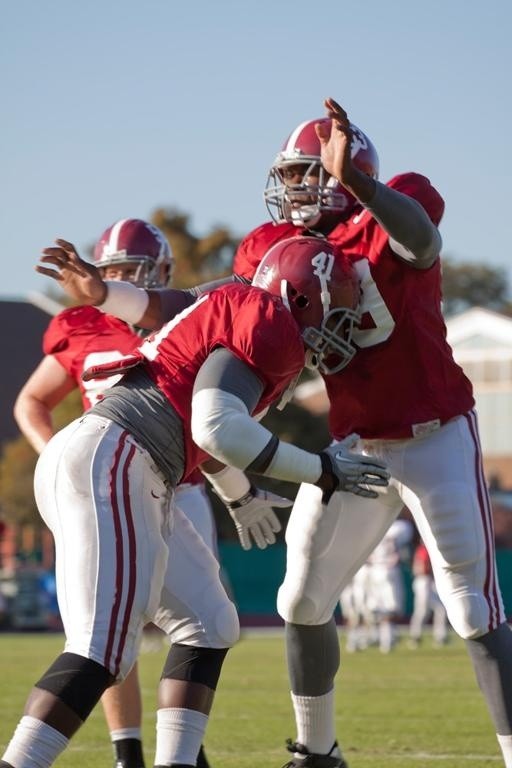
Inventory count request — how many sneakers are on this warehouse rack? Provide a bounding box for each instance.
[280,738,348,768]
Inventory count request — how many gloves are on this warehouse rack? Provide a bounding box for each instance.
[314,432,391,503]
[211,481,294,551]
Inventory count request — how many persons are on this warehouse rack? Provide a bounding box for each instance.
[13,217,217,768]
[34,101,512,768]
[0,236,391,768]
[338,520,450,656]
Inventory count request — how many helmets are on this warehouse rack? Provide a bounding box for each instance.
[92,219,175,288]
[251,235,362,375]
[263,118,378,227]
